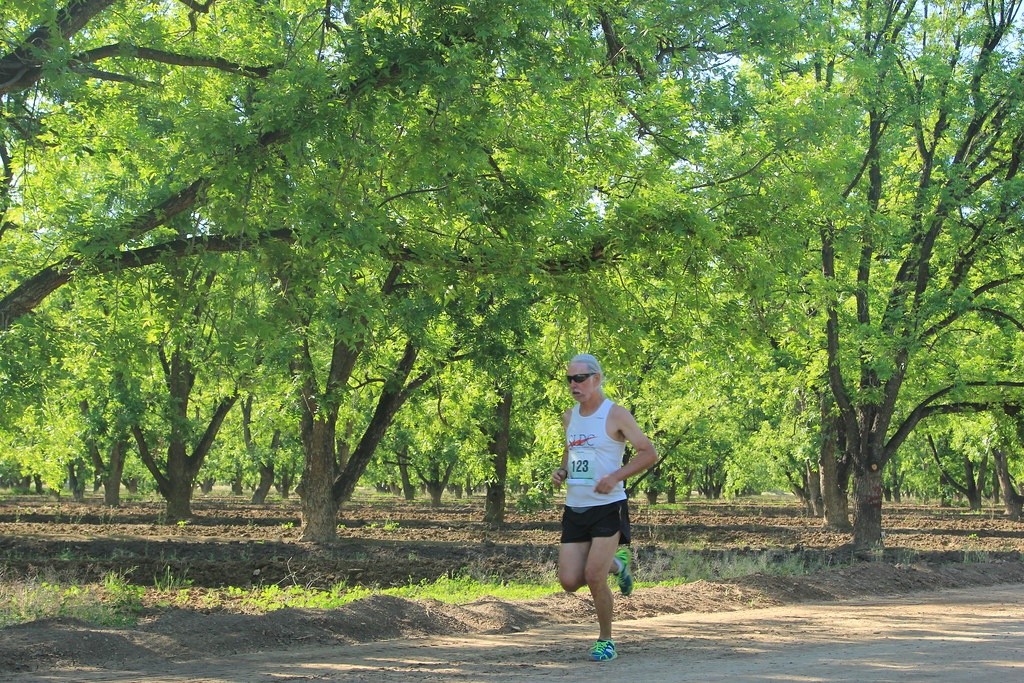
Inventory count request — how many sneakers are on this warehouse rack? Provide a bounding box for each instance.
[614,547,632,595]
[589,640,618,662]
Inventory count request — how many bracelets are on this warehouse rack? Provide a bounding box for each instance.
[562,469,567,480]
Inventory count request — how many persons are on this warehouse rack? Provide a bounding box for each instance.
[552,354,657,662]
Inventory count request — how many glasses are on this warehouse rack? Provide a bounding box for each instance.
[566,373,596,384]
